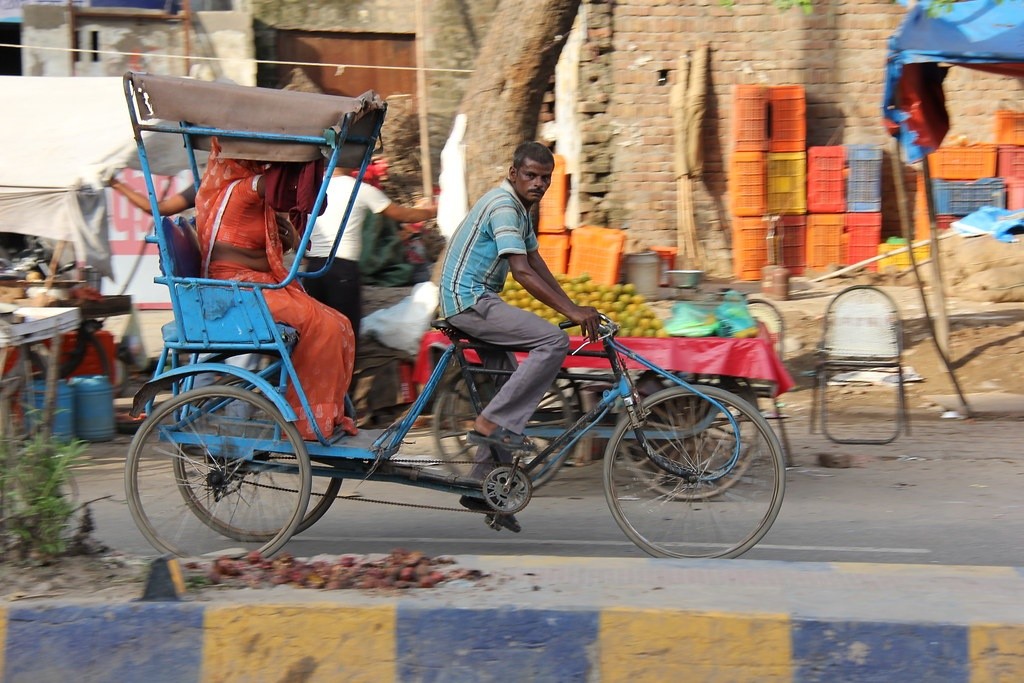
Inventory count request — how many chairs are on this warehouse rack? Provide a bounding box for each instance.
[804,283,912,445]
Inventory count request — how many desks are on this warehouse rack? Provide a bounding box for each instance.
[1,307,80,456]
[414,322,795,473]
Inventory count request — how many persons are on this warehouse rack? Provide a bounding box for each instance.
[195,133,359,442]
[305,166,439,403]
[440,138,603,535]
[107,171,204,218]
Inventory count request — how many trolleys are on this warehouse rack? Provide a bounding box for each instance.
[424,303,779,501]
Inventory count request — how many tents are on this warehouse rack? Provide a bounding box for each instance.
[0,72,163,415]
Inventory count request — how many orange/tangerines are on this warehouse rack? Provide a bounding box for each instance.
[494,270,669,339]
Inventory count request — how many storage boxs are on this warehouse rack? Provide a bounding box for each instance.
[728,78,1024,287]
[541,148,626,286]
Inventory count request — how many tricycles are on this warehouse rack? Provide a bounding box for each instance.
[120,69,786,562]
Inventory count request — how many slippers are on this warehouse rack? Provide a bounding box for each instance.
[460,495,521,533]
[466,424,537,450]
